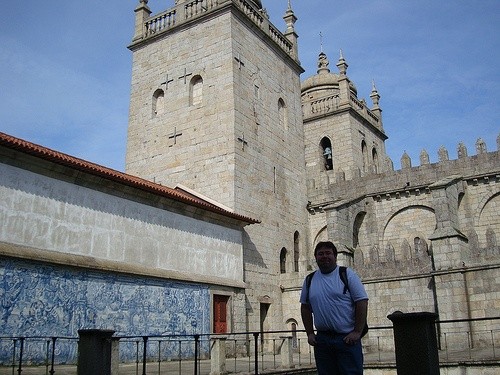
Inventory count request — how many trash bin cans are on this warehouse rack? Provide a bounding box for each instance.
[76,328,115,375]
[386,310,441,375]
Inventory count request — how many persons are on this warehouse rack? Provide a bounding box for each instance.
[299,241,369,375]
